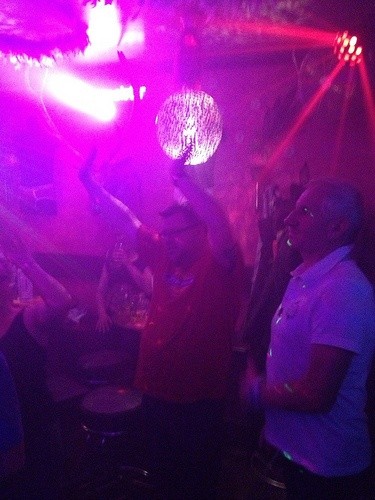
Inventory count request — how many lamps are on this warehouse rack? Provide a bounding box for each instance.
[157,84,224,164]
[333,30,365,69]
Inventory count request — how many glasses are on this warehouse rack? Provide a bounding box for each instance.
[294,202,337,225]
[158,224,192,242]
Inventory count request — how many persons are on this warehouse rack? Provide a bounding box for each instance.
[0,230,71,466]
[95,226,153,373]
[77,144,234,443]
[242,178,374,499]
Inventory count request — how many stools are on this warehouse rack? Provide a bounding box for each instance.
[80,384,153,485]
